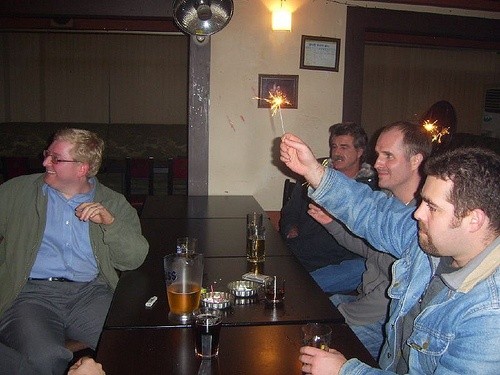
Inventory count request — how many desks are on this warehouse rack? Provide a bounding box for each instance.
[94,195,382,375]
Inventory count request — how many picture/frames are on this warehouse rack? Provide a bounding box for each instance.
[258,73,299,109]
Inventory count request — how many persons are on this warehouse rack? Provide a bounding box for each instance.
[0,130,150,375]
[279,120,433,363]
[0,341,105,375]
[280,134,500,375]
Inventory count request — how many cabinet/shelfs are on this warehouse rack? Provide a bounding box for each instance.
[0,15,188,126]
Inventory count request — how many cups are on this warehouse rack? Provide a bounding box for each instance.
[192,307,223,359]
[301,322,332,352]
[176,236,200,254]
[164,252,204,324]
[245,212,266,263]
[263,275,286,305]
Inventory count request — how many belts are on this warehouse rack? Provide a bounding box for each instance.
[29,276,74,282]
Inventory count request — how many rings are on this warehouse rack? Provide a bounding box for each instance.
[95,206,98,210]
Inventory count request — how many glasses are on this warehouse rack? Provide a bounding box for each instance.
[43,150,82,164]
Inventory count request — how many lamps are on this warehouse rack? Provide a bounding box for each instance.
[172,0,234,42]
[271,7,293,32]
